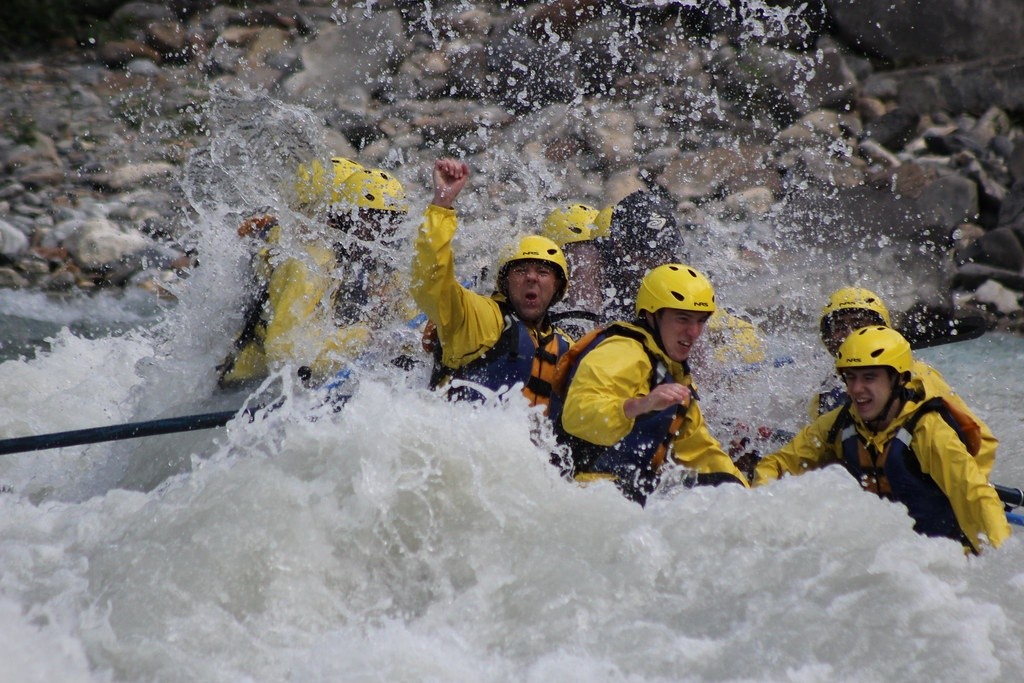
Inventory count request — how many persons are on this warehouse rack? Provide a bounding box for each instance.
[222,157,426,383]
[410,157,749,493]
[750,288,1010,554]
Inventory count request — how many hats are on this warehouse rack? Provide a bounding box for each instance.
[610,187,684,252]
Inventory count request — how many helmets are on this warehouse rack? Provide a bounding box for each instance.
[590,206,614,241]
[819,286,894,356]
[296,156,364,205]
[541,203,600,249]
[497,235,568,306]
[835,325,913,381]
[338,168,409,213]
[636,264,716,318]
